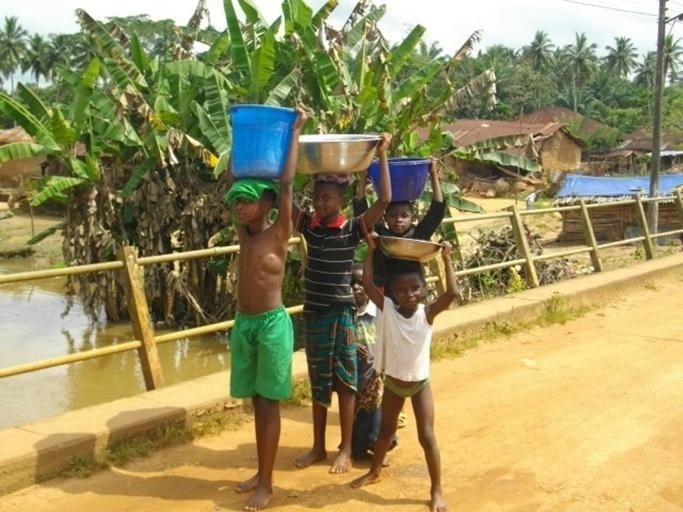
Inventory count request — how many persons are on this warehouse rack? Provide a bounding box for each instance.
[525,189,543,210]
[274,133,392,472]
[352,156,447,428]
[229,108,308,510]
[349,233,461,512]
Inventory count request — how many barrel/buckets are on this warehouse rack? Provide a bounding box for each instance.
[228,104,297,179]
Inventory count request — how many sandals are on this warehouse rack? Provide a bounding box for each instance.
[397,414,407,428]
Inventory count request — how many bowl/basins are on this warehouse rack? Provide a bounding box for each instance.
[299,134,381,176]
[370,234,443,263]
